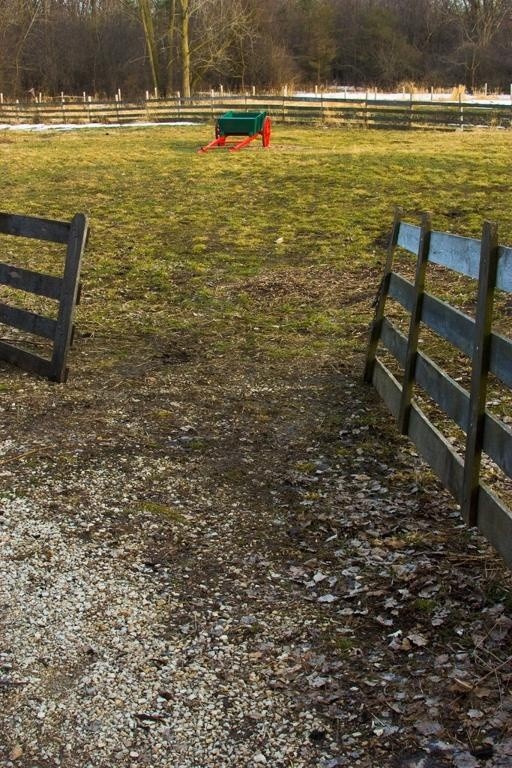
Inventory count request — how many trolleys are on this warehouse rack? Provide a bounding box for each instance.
[196,110,271,154]
